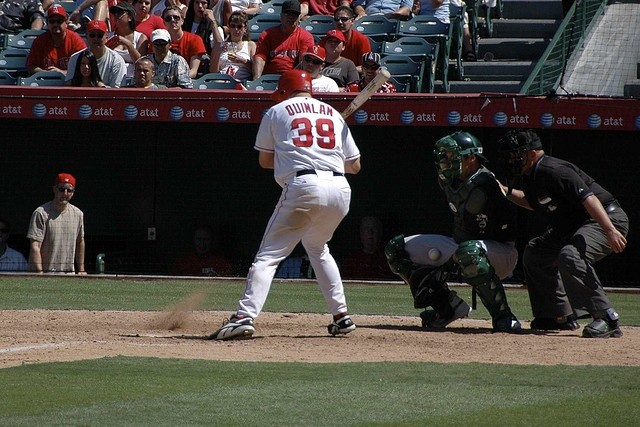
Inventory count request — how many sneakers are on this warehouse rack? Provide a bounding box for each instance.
[327,316,356,337]
[209,314,255,339]
[583,307,623,338]
[420,296,470,331]
[531,312,580,330]
[492,313,521,331]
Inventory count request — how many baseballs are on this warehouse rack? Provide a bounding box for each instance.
[428,248,442,262]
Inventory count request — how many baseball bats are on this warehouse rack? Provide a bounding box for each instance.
[341,69,390,121]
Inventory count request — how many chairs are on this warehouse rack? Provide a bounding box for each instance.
[0,0,113,87]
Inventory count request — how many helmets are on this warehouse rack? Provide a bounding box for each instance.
[272,69,312,102]
[427,130,488,202]
[498,129,542,196]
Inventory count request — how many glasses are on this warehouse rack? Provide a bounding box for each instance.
[333,17,352,22]
[112,11,127,15]
[47,19,68,24]
[55,186,74,192]
[228,23,247,29]
[153,41,169,46]
[163,16,180,22]
[78,61,92,67]
[87,32,105,38]
[0,228,11,234]
[362,63,378,70]
[283,11,299,17]
[304,55,322,65]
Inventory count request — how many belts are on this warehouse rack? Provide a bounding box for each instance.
[605,198,619,213]
[296,169,343,177]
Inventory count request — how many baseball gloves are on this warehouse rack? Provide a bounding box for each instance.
[408,266,450,309]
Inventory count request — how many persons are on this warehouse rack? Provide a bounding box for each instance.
[26,173,87,275]
[160,0,188,22]
[145,29,193,89]
[65,21,128,87]
[182,1,223,71]
[349,0,413,23]
[208,0,233,42]
[276,248,316,279]
[412,0,476,62]
[319,6,372,72]
[159,6,207,78]
[67,0,121,31]
[172,225,224,277]
[0,221,27,272]
[207,69,362,339]
[336,215,403,281]
[297,0,349,22]
[26,3,87,73]
[385,130,519,333]
[131,0,168,36]
[130,56,167,89]
[493,129,629,339]
[323,29,359,92]
[209,11,256,82]
[64,50,107,87]
[0,0,45,49]
[230,0,263,16]
[234,45,340,91]
[41,0,54,15]
[345,51,397,94]
[413,0,420,16]
[105,2,148,78]
[253,1,314,81]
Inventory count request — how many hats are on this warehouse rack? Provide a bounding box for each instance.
[86,20,108,33]
[281,1,301,14]
[323,29,345,43]
[150,29,171,42]
[109,2,136,15]
[303,44,327,61]
[55,173,76,188]
[362,51,381,66]
[48,4,66,18]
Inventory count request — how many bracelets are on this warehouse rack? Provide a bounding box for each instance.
[507,185,513,198]
[210,19,216,27]
[246,59,250,66]
